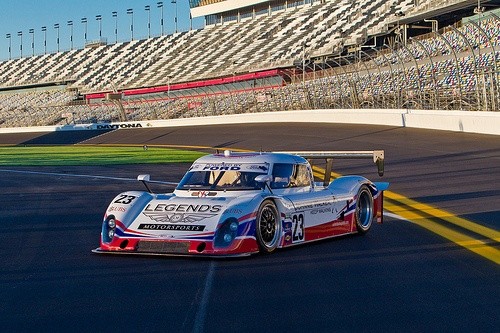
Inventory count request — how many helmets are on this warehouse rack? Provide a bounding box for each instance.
[240,172,261,186]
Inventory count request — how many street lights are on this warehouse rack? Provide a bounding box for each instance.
[111,11,117,43]
[17,30,23,59]
[157,2,164,36]
[170,0,177,33]
[126,8,133,41]
[66,20,73,51]
[54,23,59,52]
[166,75,172,116]
[95,14,102,43]
[81,17,87,44]
[41,25,46,55]
[144,5,151,39]
[6,33,11,60]
[29,28,35,57]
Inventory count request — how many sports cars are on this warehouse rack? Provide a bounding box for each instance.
[90,149,391,258]
